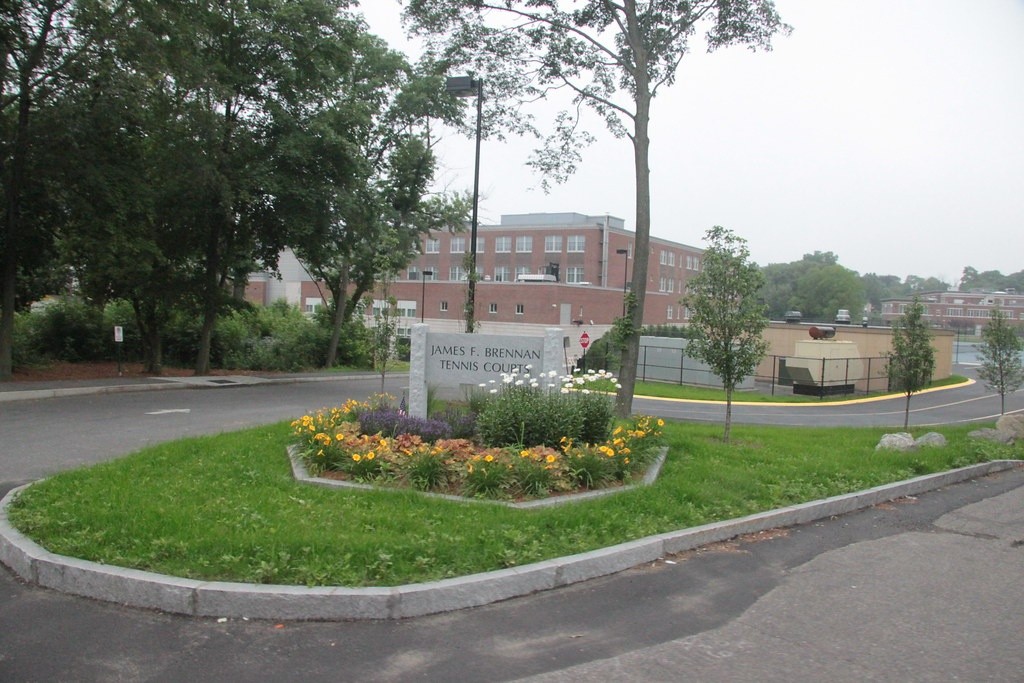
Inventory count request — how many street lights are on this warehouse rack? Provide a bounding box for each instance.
[421,269,432,320]
[447,75,483,332]
[616,248,629,319]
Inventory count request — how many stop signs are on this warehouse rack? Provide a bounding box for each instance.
[579,333,590,349]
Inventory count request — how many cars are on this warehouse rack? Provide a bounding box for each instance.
[834,310,851,324]
